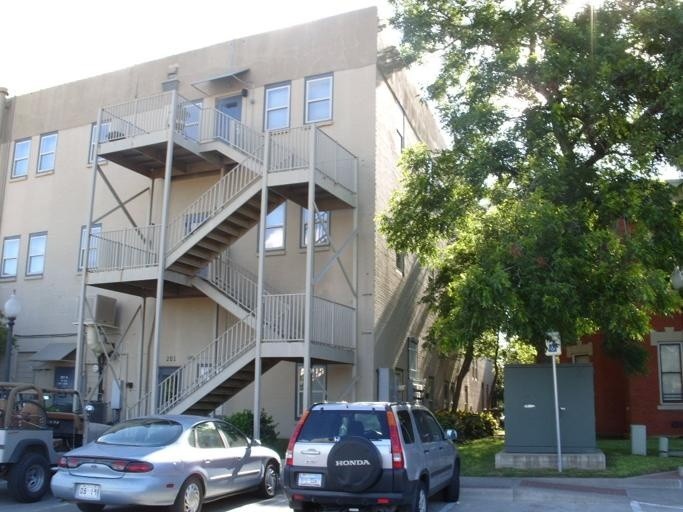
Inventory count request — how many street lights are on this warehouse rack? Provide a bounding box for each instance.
[5,290,20,382]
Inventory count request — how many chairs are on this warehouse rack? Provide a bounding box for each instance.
[0,399,18,431]
[20,400,62,447]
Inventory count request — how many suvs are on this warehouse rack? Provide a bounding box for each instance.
[0,382,113,503]
[284,402,460,512]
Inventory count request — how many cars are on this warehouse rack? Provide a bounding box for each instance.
[51,415,282,512]
